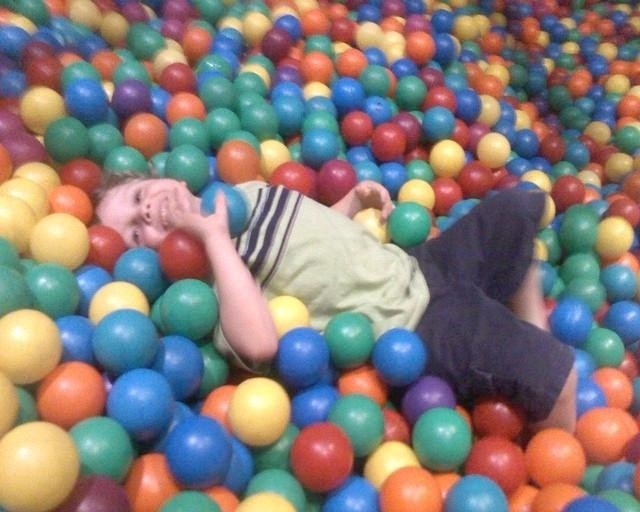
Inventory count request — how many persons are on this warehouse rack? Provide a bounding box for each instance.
[91,174,576,441]
